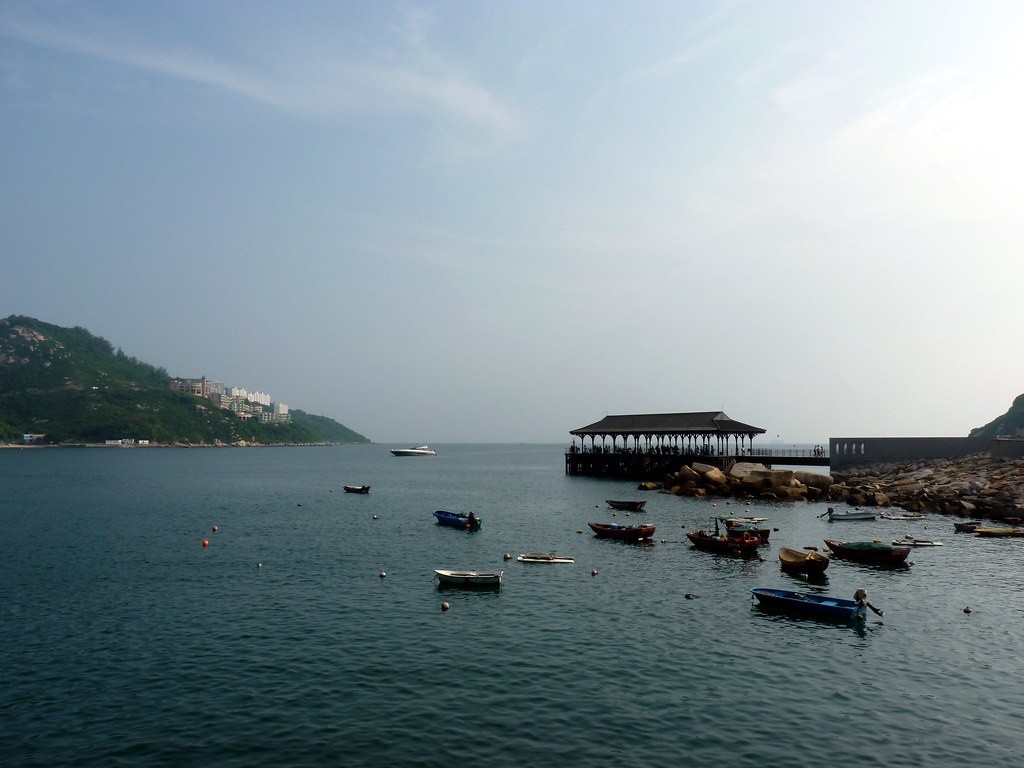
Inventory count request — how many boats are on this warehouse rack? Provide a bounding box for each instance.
[432,569,504,589]
[431,509,481,531]
[390,445,436,455]
[750,586,884,628]
[518,551,574,566]
[952,521,981,529]
[893,535,944,547]
[820,507,877,521]
[343,484,371,496]
[974,525,1024,534]
[778,546,830,573]
[686,516,763,556]
[605,500,647,511]
[726,522,770,542]
[824,538,910,564]
[882,512,923,520]
[589,520,655,540]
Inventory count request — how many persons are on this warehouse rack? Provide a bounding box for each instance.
[812,445,826,457]
[570,444,715,456]
[741,446,746,454]
[718,520,728,540]
